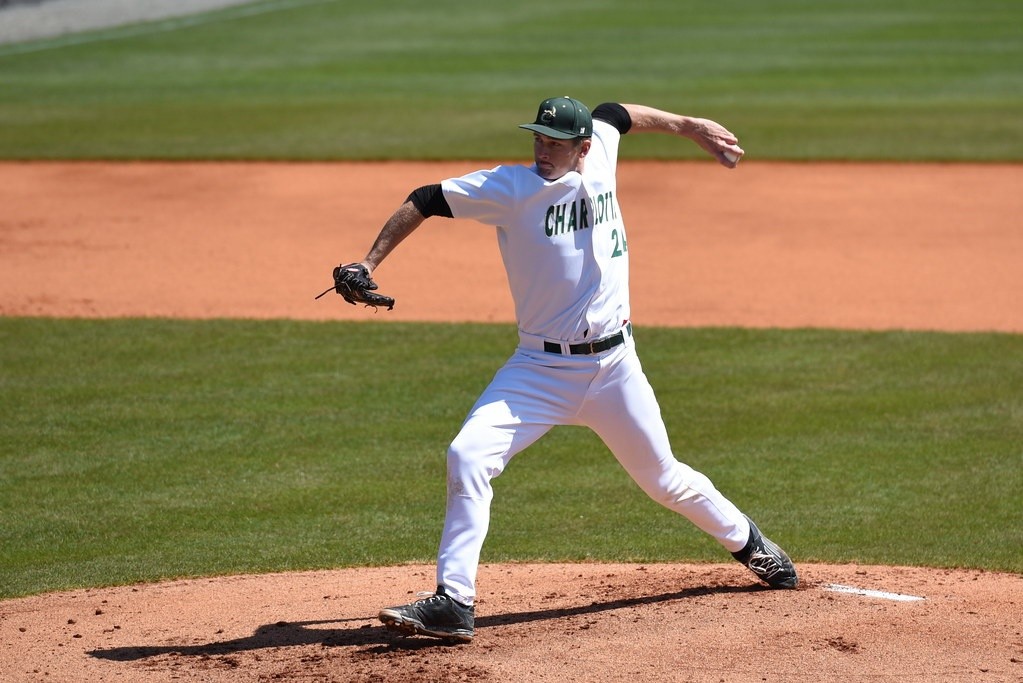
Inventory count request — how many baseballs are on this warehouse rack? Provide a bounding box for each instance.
[724,144,741,164]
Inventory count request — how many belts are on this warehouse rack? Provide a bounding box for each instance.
[544,322,633,354]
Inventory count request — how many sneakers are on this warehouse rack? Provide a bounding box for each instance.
[378,584,475,641]
[730,512,798,590]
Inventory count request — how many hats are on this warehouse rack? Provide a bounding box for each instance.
[517,95,593,139]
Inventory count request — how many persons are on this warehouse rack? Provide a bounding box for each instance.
[332,94,803,635]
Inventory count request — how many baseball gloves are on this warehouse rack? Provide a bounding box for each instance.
[315,262,395,314]
